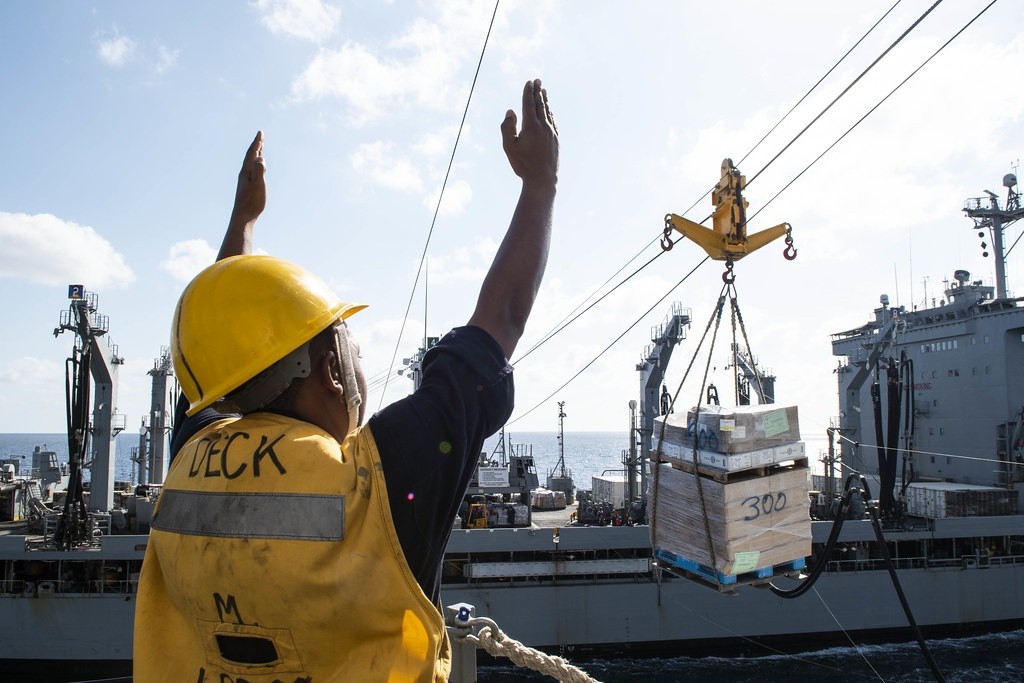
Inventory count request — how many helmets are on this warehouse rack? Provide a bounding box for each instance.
[170,255,369,418]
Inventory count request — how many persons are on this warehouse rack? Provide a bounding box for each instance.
[131,79,560,683]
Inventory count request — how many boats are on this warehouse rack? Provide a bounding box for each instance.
[0,172,1024,683]
